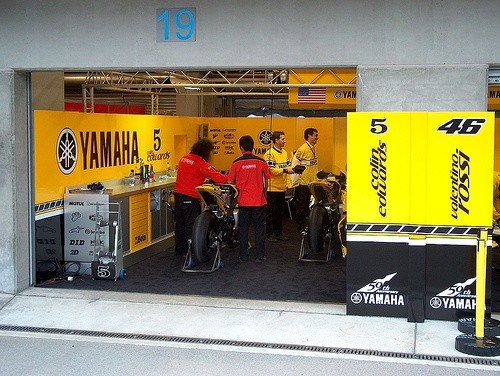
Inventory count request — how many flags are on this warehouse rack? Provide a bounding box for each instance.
[297,87,326,104]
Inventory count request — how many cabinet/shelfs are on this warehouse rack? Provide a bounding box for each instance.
[65,170,176,269]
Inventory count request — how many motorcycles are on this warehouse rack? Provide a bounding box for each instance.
[307,170,347,253]
[191,169,238,263]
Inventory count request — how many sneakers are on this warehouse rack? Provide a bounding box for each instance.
[298,224,306,233]
[240,256,249,263]
[265,233,277,242]
[256,255,267,263]
[276,234,289,241]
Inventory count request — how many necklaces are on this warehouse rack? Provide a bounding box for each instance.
[306,142,315,157]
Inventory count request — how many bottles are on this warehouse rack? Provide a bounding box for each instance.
[150,164,154,181]
[166,161,171,176]
[175,164,178,176]
[129,169,134,177]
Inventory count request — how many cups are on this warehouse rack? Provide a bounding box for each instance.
[122,176,140,186]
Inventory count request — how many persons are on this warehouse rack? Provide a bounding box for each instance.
[294,128,319,238]
[228,135,274,264]
[263,130,295,242]
[173,138,228,266]
